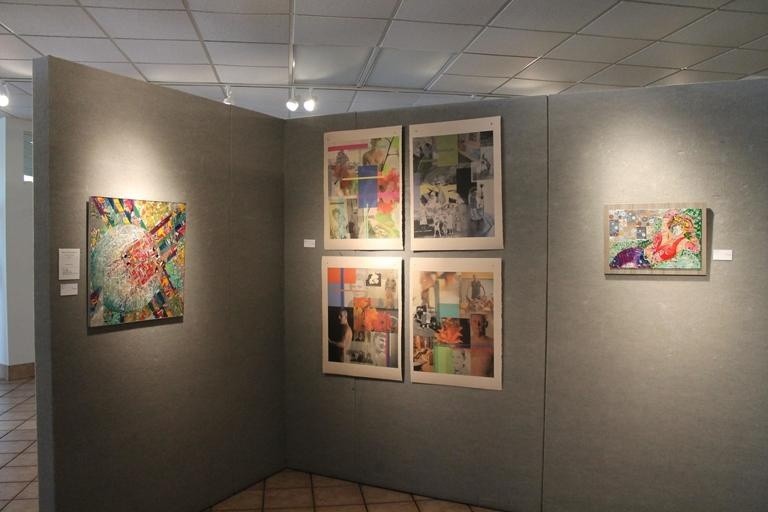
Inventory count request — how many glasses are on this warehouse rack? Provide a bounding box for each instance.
[0,82,11,107]
[285,87,317,112]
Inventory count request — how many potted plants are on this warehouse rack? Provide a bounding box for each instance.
[603,202,708,277]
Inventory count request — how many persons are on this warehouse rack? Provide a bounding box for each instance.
[327,270,493,378]
[328,132,495,240]
